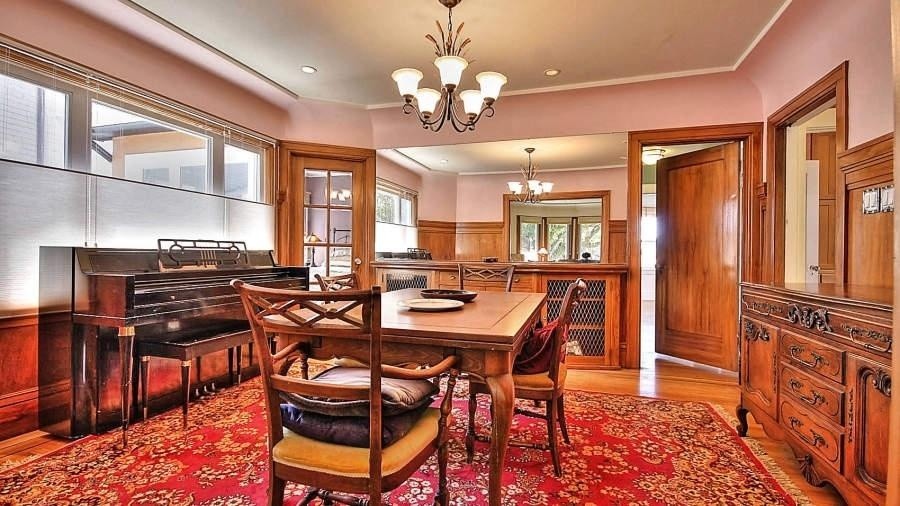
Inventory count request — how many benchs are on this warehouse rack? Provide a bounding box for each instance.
[137,319,277,431]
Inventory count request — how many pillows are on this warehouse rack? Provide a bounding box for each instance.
[283,368,440,418]
[333,249,345,256]
[278,398,436,449]
[511,317,570,374]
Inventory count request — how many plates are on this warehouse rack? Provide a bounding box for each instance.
[402,299,466,312]
[420,289,477,302]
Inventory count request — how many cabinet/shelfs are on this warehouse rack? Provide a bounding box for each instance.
[433,268,541,293]
[736,280,892,506]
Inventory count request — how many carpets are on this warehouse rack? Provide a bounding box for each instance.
[0,355,822,505]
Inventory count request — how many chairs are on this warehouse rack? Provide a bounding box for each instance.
[458,264,516,292]
[231,279,458,505]
[316,272,361,305]
[465,277,589,478]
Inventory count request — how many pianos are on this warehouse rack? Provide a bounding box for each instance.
[37,247,310,439]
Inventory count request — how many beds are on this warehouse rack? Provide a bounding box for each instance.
[306,228,352,291]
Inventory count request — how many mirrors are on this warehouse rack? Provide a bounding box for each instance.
[502,189,610,264]
[374,131,629,265]
[766,59,848,296]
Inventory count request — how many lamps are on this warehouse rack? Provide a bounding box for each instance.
[390,1,508,133]
[305,232,322,266]
[506,147,554,204]
[538,248,548,261]
